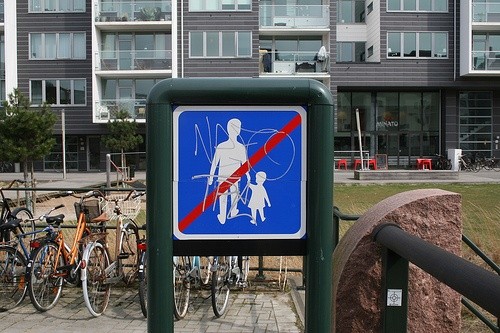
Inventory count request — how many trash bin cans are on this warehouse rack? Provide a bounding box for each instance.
[15,163,21,173]
[447,148,462,170]
[127,164,135,177]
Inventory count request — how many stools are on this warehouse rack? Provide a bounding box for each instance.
[337,160,348,170]
[354,159,362,170]
[422,159,432,170]
[416,159,422,170]
[367,159,377,170]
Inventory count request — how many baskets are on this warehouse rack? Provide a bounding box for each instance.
[74,198,104,222]
[100,194,141,221]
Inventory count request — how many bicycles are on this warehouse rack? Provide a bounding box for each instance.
[434,153,496,173]
[0,180,250,319]
